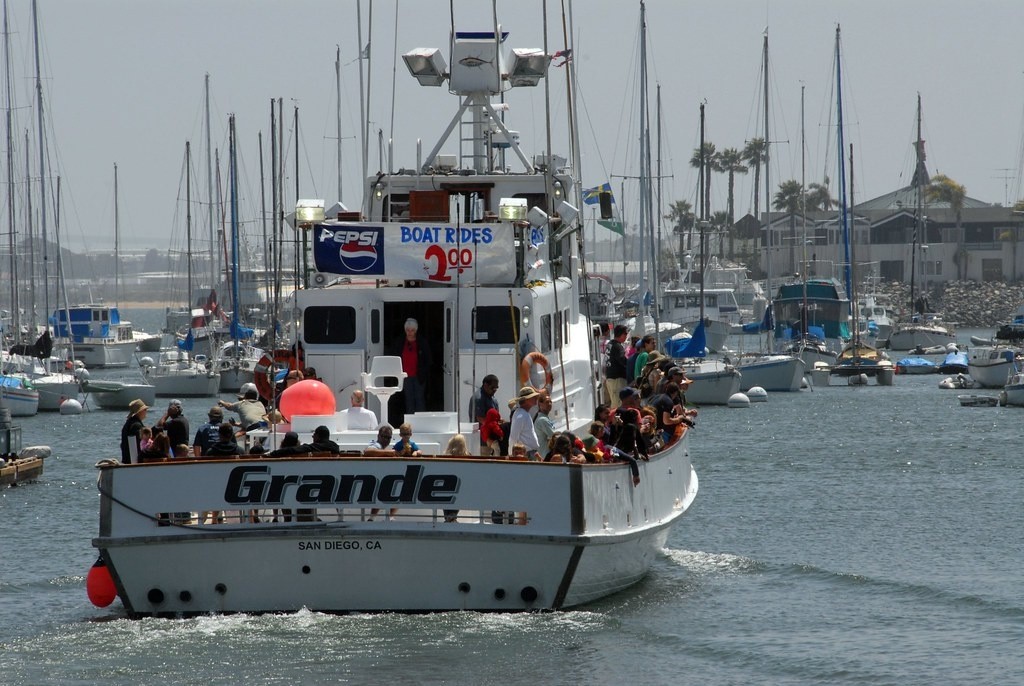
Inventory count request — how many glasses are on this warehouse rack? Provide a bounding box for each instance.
[487,385,498,391]
[379,434,392,440]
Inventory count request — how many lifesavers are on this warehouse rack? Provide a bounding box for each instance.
[522,351,554,395]
[253,349,304,402]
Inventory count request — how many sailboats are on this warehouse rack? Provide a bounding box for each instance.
[0,2,1023,476]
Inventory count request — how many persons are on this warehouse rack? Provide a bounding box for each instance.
[120,322,697,465]
[396,318,429,412]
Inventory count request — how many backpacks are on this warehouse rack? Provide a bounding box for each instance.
[640,366,655,397]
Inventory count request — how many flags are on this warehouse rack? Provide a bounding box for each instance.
[598,220,624,235]
[581,183,615,205]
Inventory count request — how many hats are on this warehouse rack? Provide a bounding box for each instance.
[167,399,183,420]
[285,431,298,446]
[126,399,149,418]
[619,386,639,401]
[635,339,642,349]
[217,424,234,435]
[235,383,259,401]
[404,318,418,332]
[667,366,686,377]
[646,350,665,365]
[515,387,540,401]
[208,406,225,419]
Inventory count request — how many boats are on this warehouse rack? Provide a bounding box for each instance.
[907,345,946,355]
[87,26,701,619]
[895,357,935,374]
[87,371,156,408]
[996,314,1023,341]
[966,342,1022,385]
[1003,374,1023,406]
[958,392,1000,407]
[939,375,965,390]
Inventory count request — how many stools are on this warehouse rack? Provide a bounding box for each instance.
[361,356,408,431]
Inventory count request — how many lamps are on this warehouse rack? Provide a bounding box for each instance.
[295,199,325,221]
[375,183,384,201]
[498,197,528,222]
[522,305,530,327]
[292,308,300,331]
[402,49,450,87]
[502,47,552,87]
[554,181,562,200]
